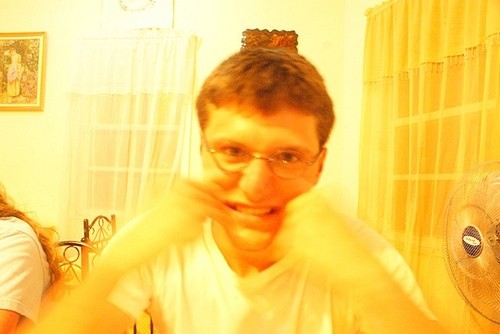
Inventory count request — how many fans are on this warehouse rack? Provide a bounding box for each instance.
[443,160,499,324]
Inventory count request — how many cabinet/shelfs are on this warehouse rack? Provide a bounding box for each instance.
[68,36,197,221]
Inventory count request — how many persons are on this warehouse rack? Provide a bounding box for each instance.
[0,185,62,333]
[24,48,450,333]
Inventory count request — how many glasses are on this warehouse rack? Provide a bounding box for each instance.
[202,133,323,180]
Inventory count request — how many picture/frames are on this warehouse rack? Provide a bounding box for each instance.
[0,30,46,111]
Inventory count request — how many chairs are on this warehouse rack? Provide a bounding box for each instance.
[81,214,154,333]
[50,241,107,300]
